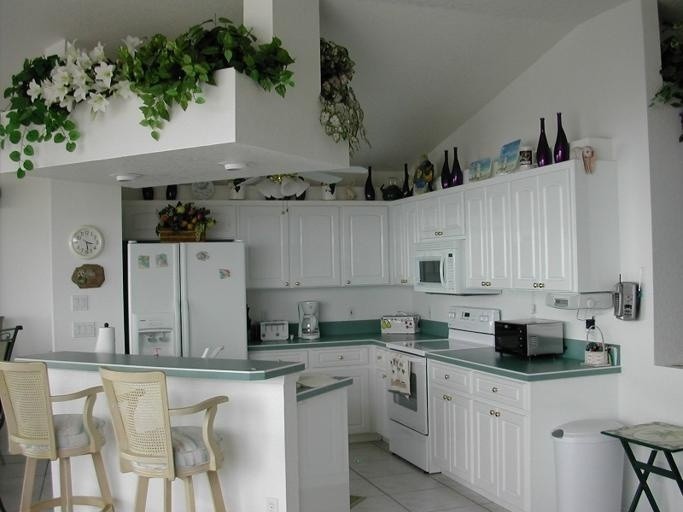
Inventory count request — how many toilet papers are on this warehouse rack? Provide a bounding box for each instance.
[95,328,115,354]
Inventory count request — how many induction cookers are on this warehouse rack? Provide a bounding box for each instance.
[385,330,496,358]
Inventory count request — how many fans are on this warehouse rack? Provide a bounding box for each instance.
[236,171,343,188]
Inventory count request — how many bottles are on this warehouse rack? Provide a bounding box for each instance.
[531,110,569,167]
[401,162,412,197]
[363,163,376,202]
[437,144,462,190]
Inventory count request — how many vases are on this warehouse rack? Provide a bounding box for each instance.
[554,112,568,163]
[365,166,375,200]
[441,147,463,189]
[536,118,552,167]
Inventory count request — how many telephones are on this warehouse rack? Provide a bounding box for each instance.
[612,274,640,321]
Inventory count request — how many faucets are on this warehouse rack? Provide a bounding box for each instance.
[207,343,226,361]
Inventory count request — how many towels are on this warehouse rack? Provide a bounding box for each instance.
[385,353,411,400]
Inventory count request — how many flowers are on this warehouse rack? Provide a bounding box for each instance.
[0,12,144,179]
[316,33,372,156]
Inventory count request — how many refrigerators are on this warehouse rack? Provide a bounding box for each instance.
[124,237,247,359]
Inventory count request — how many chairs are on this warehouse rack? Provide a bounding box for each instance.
[0,361,116,512]
[0,325,23,512]
[96,365,230,512]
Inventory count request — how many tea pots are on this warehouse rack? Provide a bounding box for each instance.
[380,182,403,202]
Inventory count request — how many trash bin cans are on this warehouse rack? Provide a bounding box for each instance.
[552,419,625,512]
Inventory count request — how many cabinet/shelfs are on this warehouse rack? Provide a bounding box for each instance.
[392,160,617,293]
[428,358,620,511]
[122,199,392,289]
[248,345,370,443]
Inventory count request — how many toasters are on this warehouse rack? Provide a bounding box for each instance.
[260,320,289,342]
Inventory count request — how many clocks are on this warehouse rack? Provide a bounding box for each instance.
[68,225,104,259]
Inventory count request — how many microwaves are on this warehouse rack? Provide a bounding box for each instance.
[411,248,502,296]
[495,317,565,359]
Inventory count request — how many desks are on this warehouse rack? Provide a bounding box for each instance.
[601,419,683,512]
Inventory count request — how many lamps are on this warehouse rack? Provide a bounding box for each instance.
[256,172,311,199]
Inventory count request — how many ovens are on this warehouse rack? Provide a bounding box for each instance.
[385,349,429,436]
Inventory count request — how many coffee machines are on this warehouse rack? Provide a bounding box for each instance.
[297,300,320,339]
